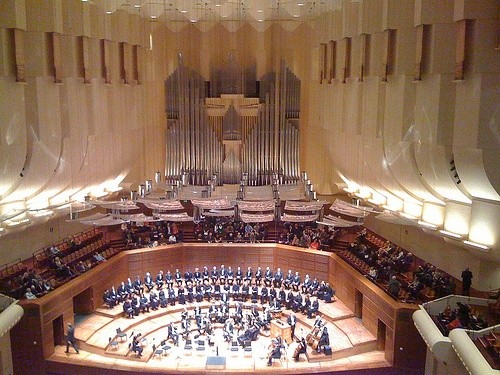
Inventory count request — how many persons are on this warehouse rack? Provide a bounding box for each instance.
[0,213,491,368]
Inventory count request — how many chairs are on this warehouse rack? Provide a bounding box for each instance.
[474,332,500,370]
[0,257,54,301]
[277,221,340,251]
[433,303,482,336]
[105,269,336,367]
[121,221,184,249]
[32,226,119,281]
[193,211,268,243]
[337,232,442,303]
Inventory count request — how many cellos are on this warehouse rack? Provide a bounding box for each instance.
[121,299,327,366]
